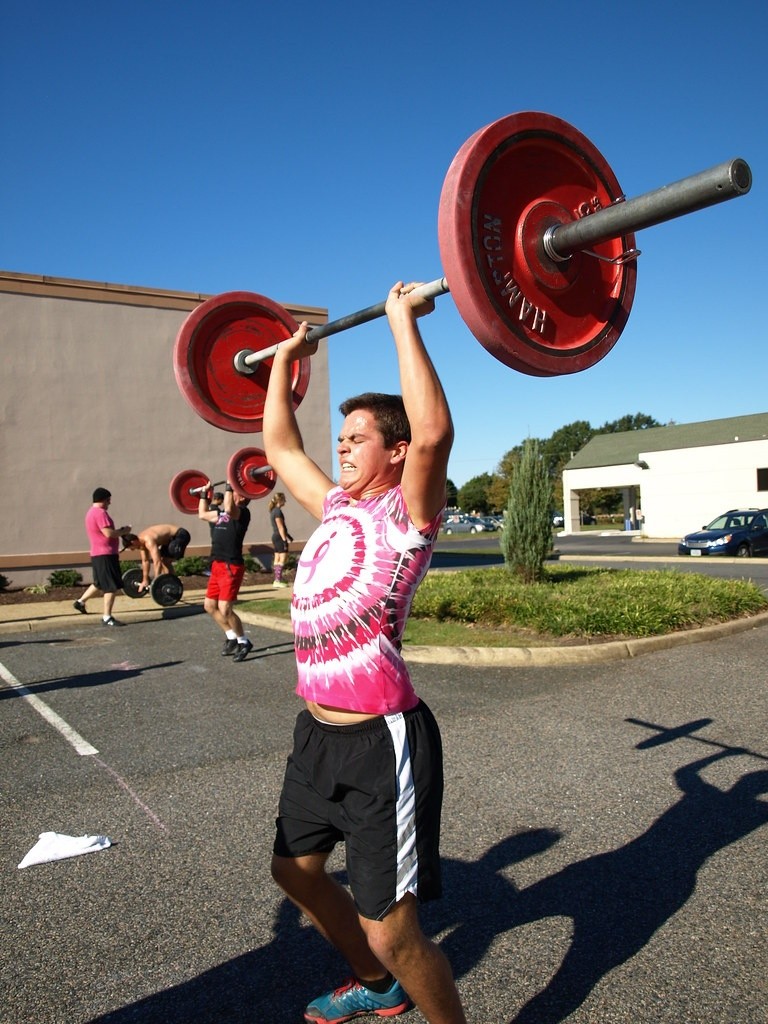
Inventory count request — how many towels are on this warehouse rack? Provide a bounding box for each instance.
[16,831,113,873]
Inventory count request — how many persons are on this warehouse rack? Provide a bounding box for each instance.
[123,524,191,593]
[72,487,127,626]
[268,493,294,588]
[198,478,253,664]
[261,277,471,1024]
[207,492,225,544]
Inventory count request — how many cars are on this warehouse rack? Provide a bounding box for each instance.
[559,513,597,525]
[482,516,508,532]
[442,516,487,534]
[475,517,495,532]
[678,506,768,557]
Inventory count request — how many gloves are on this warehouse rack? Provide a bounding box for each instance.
[284,540,288,549]
[289,537,293,542]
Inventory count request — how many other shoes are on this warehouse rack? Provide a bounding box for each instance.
[73,601,87,613]
[202,571,211,575]
[281,580,288,583]
[273,581,288,587]
[101,618,127,626]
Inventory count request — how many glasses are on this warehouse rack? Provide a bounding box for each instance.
[107,499,110,502]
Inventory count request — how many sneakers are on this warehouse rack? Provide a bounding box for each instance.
[304,984,407,1024]
[222,639,237,655]
[234,639,253,661]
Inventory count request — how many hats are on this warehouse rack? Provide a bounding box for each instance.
[213,493,223,499]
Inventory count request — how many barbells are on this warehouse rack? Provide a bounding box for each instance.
[171,105,756,433]
[166,444,278,516]
[120,566,185,607]
